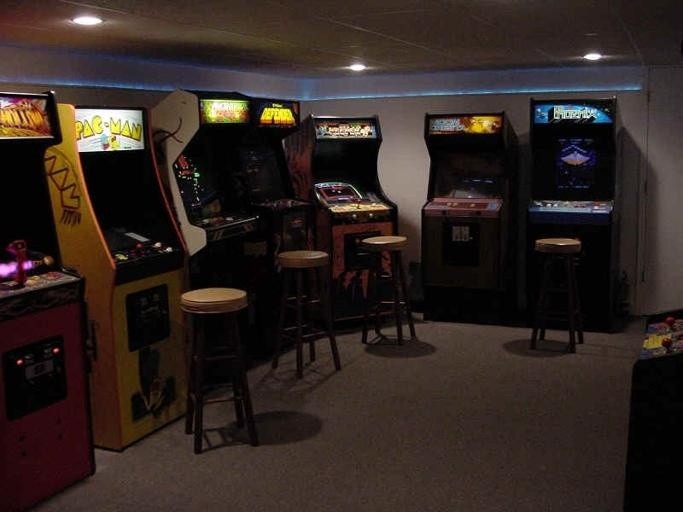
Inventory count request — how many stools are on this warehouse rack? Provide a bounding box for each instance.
[360,235,415,344]
[526,238,584,352]
[179,287,258,455]
[272,250,342,379]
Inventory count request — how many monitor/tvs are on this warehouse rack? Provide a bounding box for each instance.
[1,144,48,260]
[539,130,612,199]
[242,145,286,192]
[435,146,499,192]
[173,142,237,217]
[79,152,163,238]
[313,154,375,191]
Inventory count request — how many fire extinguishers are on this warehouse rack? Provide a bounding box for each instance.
[616,270,632,320]
